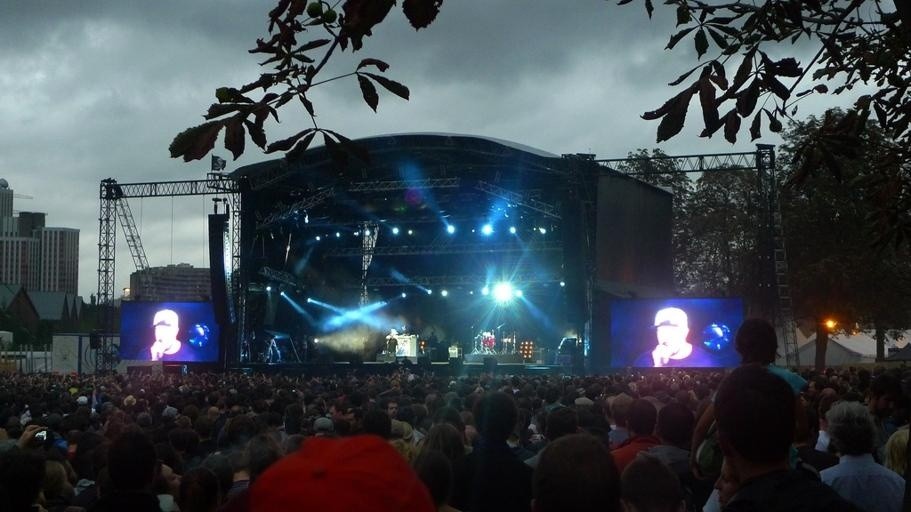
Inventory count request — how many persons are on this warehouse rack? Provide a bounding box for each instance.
[633,307,716,367]
[1,363,911,512]
[428,330,438,345]
[696,319,819,462]
[136,307,194,363]
[383,330,399,361]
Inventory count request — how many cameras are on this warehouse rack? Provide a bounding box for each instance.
[287,384,295,392]
[34,431,47,441]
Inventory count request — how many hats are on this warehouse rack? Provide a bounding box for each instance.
[123,395,136,407]
[654,308,687,327]
[152,310,178,326]
[78,396,86,405]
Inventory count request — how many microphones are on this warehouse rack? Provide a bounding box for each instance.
[663,342,669,363]
[157,339,163,358]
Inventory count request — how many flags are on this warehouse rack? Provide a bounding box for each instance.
[212,154,226,172]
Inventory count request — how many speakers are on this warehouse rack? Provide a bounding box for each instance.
[396,356,407,363]
[89,333,101,350]
[449,357,463,364]
[562,198,588,323]
[417,357,432,365]
[207,214,236,325]
[484,358,497,365]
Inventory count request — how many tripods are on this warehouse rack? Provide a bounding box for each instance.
[471,332,517,354]
